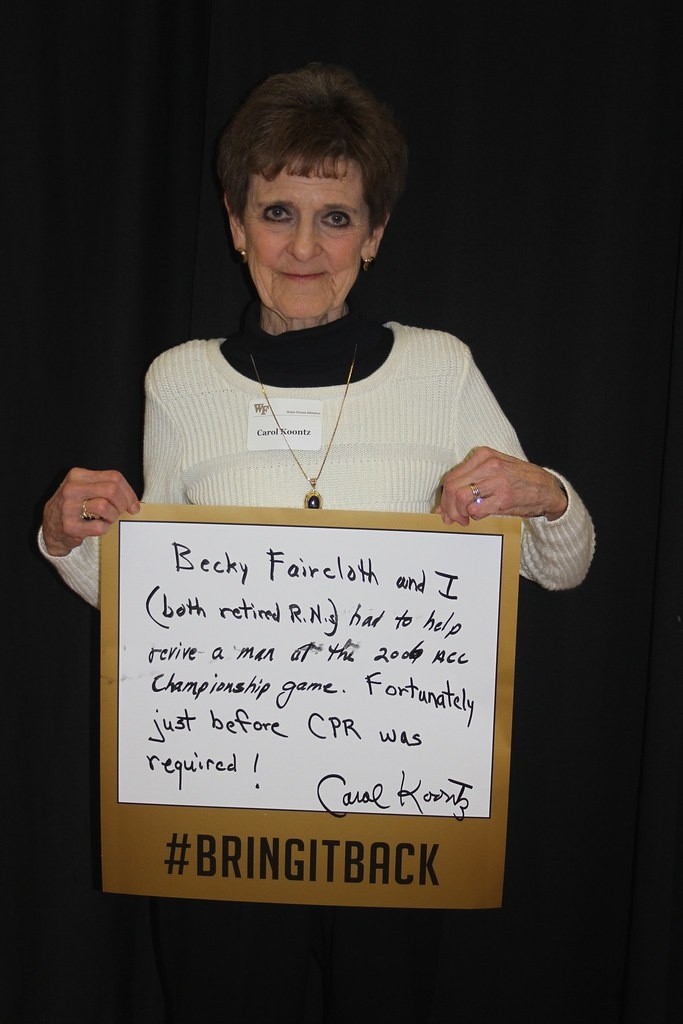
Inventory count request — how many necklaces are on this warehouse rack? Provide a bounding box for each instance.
[251,344,357,510]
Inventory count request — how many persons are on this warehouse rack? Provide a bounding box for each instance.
[31,56,594,1023]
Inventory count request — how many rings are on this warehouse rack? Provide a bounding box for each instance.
[470,483,480,500]
[80,499,96,521]
